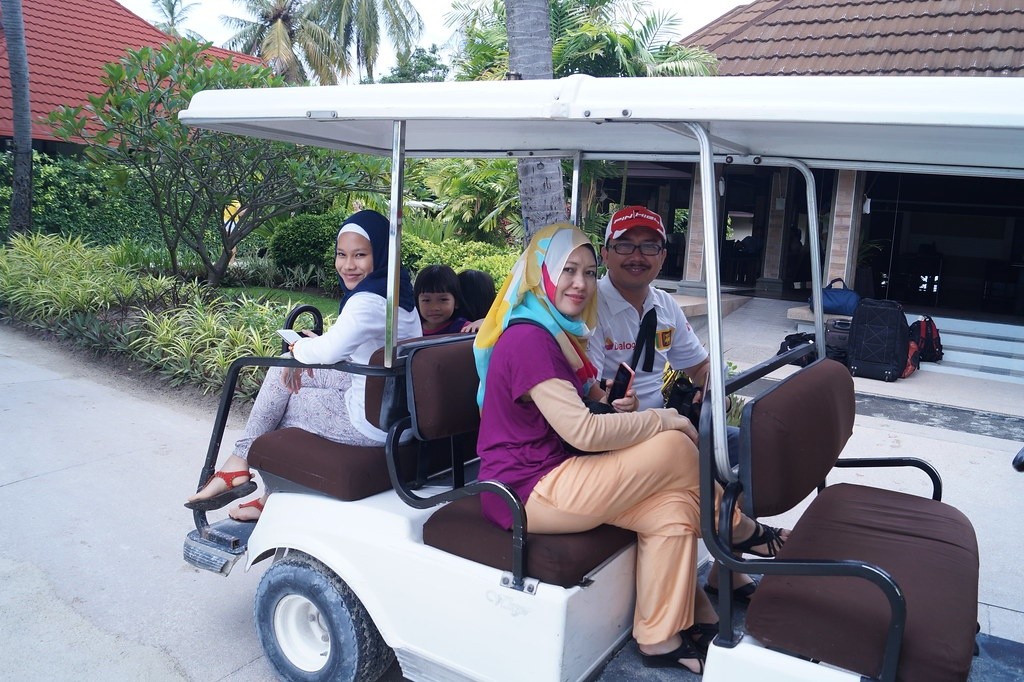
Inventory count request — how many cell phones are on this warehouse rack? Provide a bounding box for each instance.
[608,362,635,411]
[276,329,302,346]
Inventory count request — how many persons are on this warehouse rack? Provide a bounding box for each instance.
[474,224,796,675]
[584,206,761,659]
[182,208,425,524]
[413,261,498,338]
[223,199,242,267]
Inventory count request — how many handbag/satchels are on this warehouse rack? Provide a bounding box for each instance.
[777,332,818,368]
[808,278,859,316]
[667,378,701,428]
[901,339,921,378]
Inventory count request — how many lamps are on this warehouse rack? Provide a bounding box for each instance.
[718,176,726,197]
[861,193,871,214]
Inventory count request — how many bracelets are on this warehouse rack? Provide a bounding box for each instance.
[288,339,298,358]
[726,395,732,414]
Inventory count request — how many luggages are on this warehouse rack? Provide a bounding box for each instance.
[847,298,908,383]
[826,319,849,361]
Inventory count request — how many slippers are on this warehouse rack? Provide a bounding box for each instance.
[704,578,759,609]
[684,621,719,655]
[731,519,785,556]
[638,632,705,675]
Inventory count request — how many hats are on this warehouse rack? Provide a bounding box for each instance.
[605,205,667,248]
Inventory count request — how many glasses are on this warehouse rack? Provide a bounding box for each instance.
[607,242,664,256]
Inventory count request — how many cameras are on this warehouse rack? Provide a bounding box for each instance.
[666,377,696,419]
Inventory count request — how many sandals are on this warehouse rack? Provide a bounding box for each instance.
[228,497,264,522]
[184,471,258,511]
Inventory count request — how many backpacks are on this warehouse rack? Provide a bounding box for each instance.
[909,315,944,361]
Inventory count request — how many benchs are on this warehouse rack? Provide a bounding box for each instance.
[245,331,467,502]
[385,333,635,588]
[714,357,980,682]
